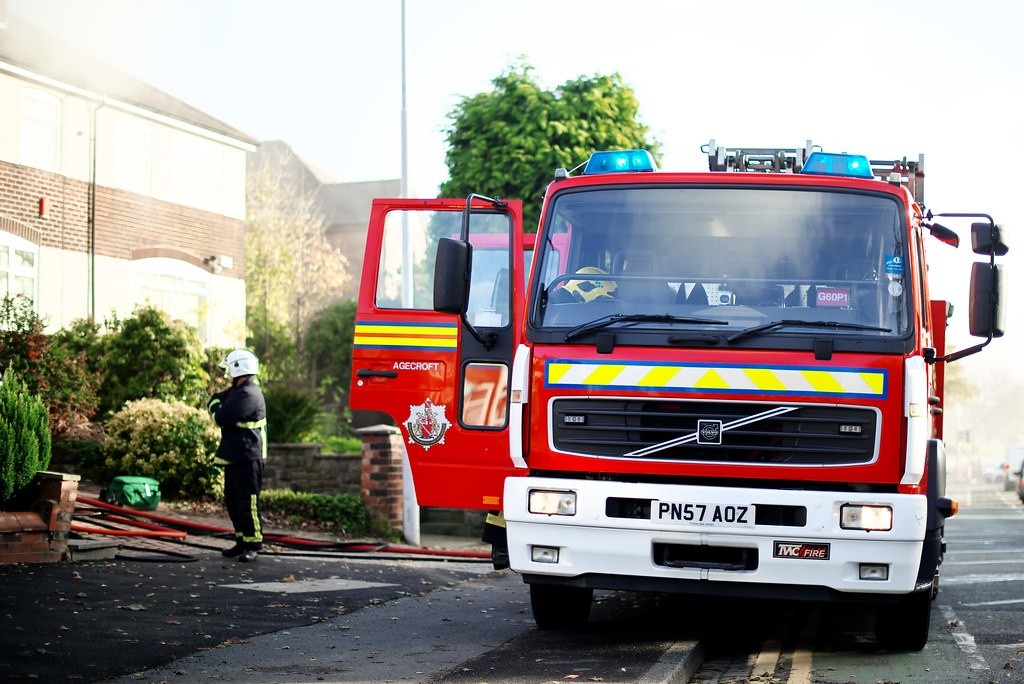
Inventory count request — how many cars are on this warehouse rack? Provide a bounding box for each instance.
[1001,457,1018,490]
[1014,460,1024,503]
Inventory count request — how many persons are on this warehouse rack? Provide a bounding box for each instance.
[207,348,268,561]
[481,265,623,571]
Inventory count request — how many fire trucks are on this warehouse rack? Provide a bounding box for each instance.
[347,137,1009,654]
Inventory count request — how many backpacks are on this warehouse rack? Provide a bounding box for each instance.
[106,476,161,510]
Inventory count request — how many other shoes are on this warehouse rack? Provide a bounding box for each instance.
[222,545,246,558]
[239,550,257,562]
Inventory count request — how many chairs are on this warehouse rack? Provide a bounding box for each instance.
[610,249,671,307]
[831,261,879,312]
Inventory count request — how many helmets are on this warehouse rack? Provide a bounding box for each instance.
[566,266,618,302]
[219,350,259,377]
